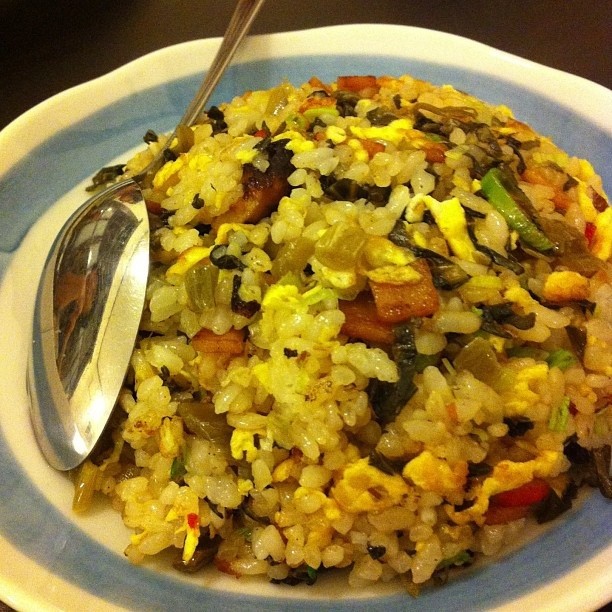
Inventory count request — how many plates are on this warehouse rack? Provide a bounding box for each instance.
[0,23,611,608]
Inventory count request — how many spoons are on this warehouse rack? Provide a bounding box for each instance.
[26,0,266,471]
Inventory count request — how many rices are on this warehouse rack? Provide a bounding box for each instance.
[72,73,611,600]
